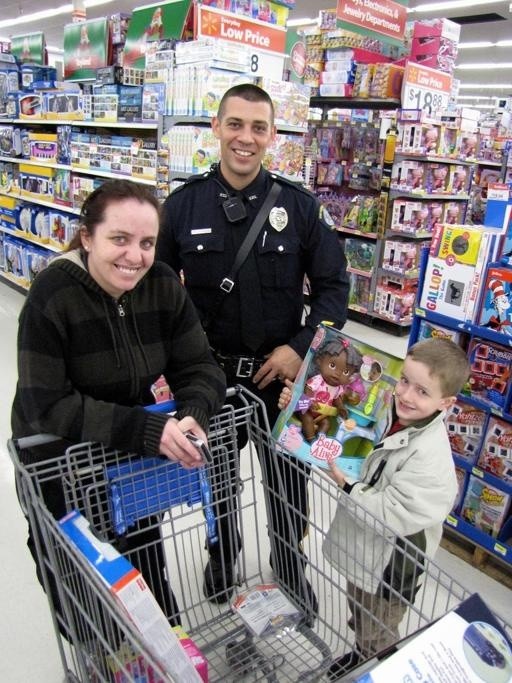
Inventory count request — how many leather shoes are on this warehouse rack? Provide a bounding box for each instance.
[202,556,236,604]
[284,576,318,618]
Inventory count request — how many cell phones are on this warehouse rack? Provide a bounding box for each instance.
[186,432,214,464]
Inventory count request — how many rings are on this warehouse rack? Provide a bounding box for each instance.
[277,376,285,381]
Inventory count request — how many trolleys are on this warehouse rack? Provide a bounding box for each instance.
[6,377,510,683]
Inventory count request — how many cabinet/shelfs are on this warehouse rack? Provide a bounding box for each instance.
[158,115,220,205]
[0,96,158,295]
[403,246,512,588]
[261,97,509,336]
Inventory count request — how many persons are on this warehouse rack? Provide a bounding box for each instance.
[9,178,227,660]
[154,82,350,620]
[276,335,473,682]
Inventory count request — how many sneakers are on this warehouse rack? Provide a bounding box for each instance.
[327,651,363,681]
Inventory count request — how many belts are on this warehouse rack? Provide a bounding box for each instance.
[214,356,267,378]
